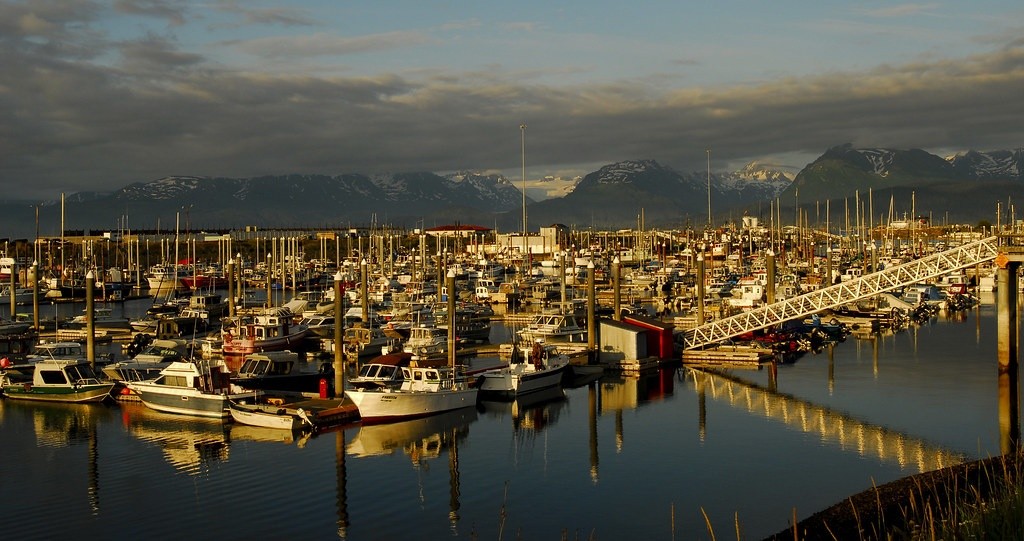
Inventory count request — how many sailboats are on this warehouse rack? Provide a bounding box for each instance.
[0,187,1024,427]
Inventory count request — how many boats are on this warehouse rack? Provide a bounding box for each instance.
[340,407,482,460]
[123,405,292,476]
[0,354,116,404]
